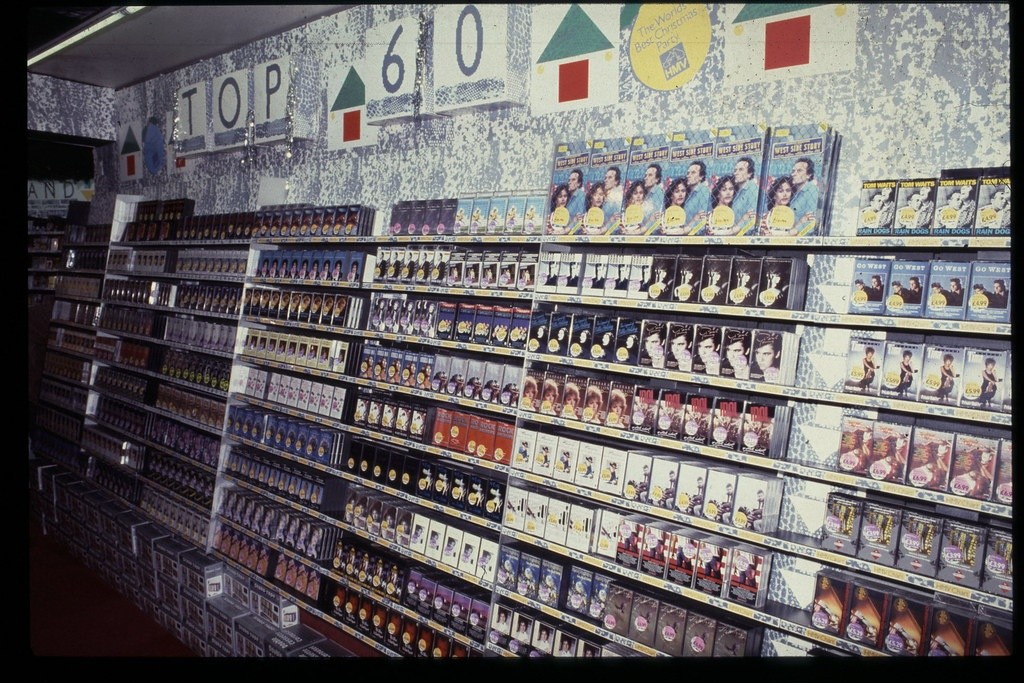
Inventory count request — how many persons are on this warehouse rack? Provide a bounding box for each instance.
[25,123,1012,657]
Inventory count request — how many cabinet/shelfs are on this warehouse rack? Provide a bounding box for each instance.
[28,196,1013,657]
